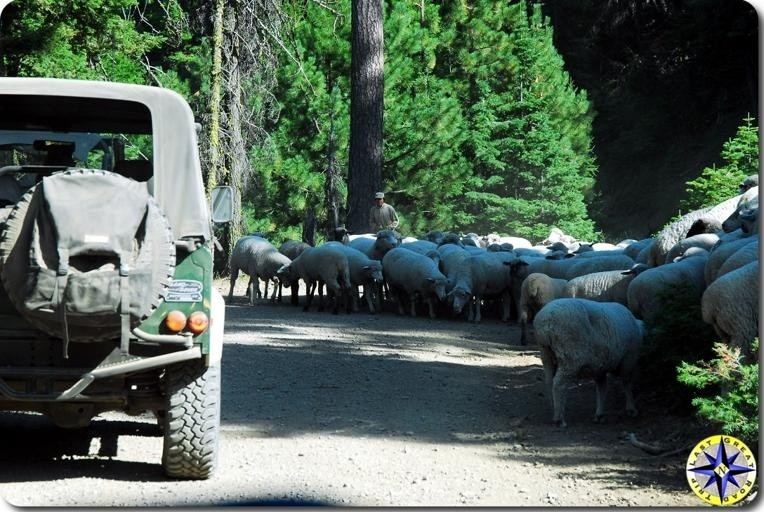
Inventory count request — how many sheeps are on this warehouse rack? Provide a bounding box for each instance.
[225,174,759,429]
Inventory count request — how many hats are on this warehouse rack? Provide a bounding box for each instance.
[374,192,384,200]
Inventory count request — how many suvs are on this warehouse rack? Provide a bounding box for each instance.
[0,77,235,477]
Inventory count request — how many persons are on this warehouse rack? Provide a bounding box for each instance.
[368,191,400,233]
